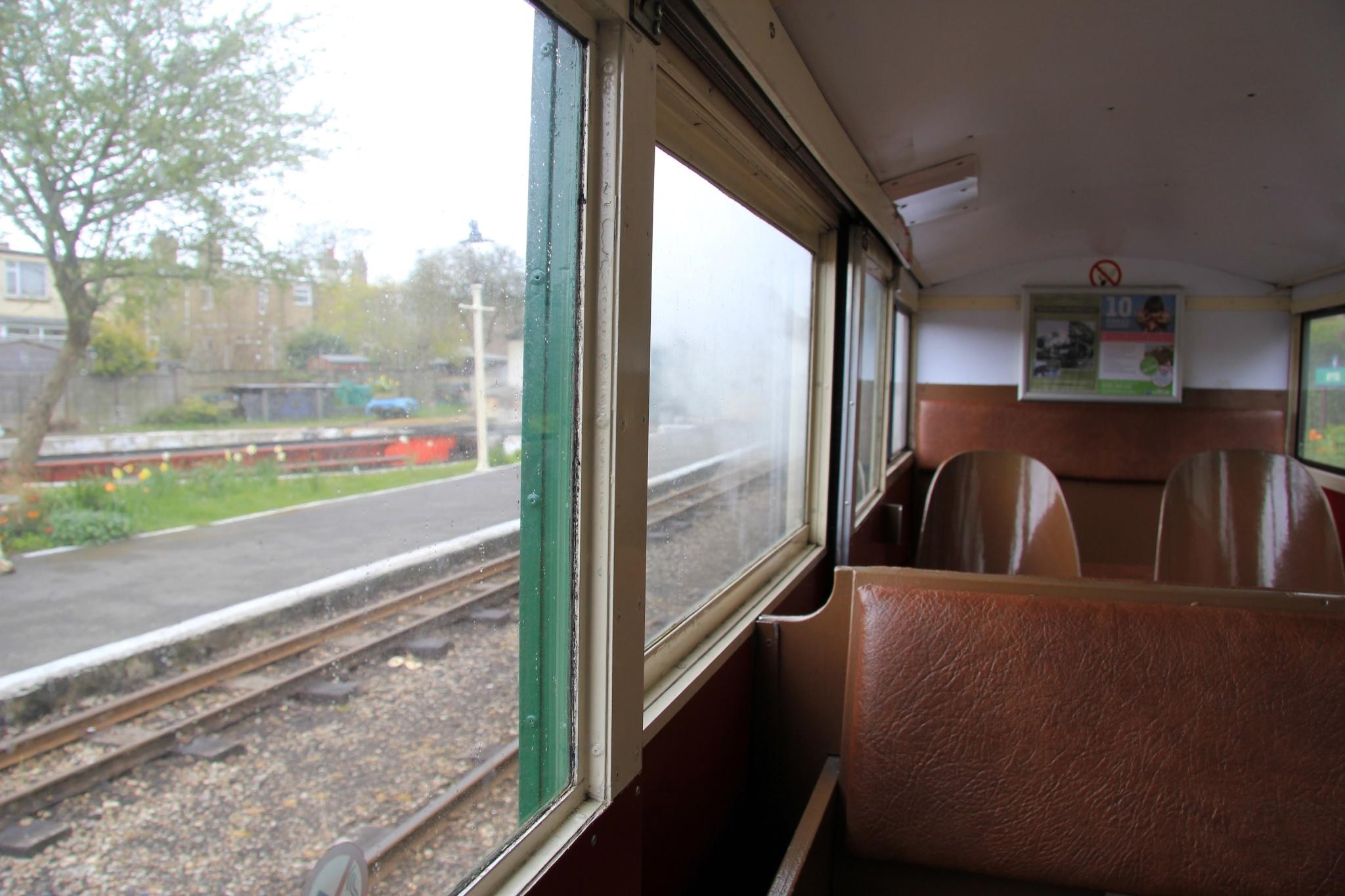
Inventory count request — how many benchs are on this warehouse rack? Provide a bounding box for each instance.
[752,565,1344,896]
[888,398,1287,581]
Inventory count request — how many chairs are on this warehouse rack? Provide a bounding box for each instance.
[915,446,1081,577]
[1152,448,1345,593]
